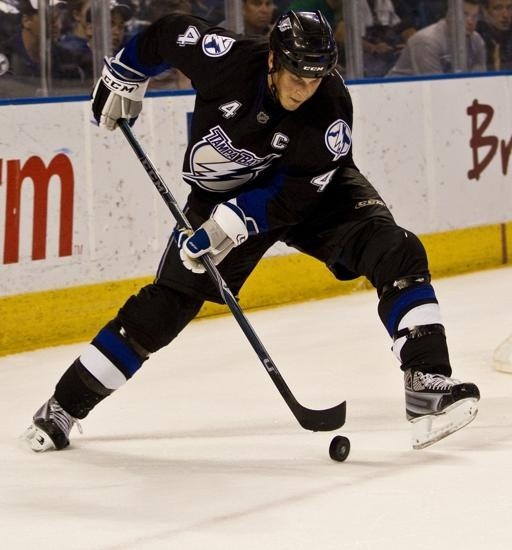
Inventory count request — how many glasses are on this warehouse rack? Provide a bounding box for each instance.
[464,10,484,20]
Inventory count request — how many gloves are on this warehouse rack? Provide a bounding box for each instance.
[179,199,261,277]
[86,44,151,133]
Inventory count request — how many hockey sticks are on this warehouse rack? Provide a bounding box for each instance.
[115,116,347,431]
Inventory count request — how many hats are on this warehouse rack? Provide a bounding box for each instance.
[86,1,130,26]
[29,0,68,16]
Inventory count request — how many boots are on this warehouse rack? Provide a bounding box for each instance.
[33,395,86,451]
[387,369,478,418]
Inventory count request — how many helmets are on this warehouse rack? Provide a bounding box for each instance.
[268,8,340,78]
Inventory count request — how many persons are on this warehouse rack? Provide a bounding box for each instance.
[31,8,481,451]
[0,0,511,102]
